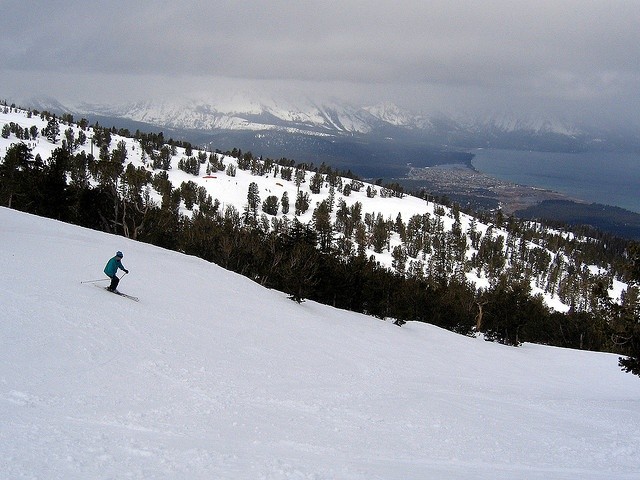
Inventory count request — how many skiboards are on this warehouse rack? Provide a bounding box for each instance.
[92,282,139,302]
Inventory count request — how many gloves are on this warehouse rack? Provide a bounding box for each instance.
[123,269,128,273]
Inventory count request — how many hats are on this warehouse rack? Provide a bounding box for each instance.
[117,251,123,257]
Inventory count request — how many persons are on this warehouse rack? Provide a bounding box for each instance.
[103,250,129,293]
